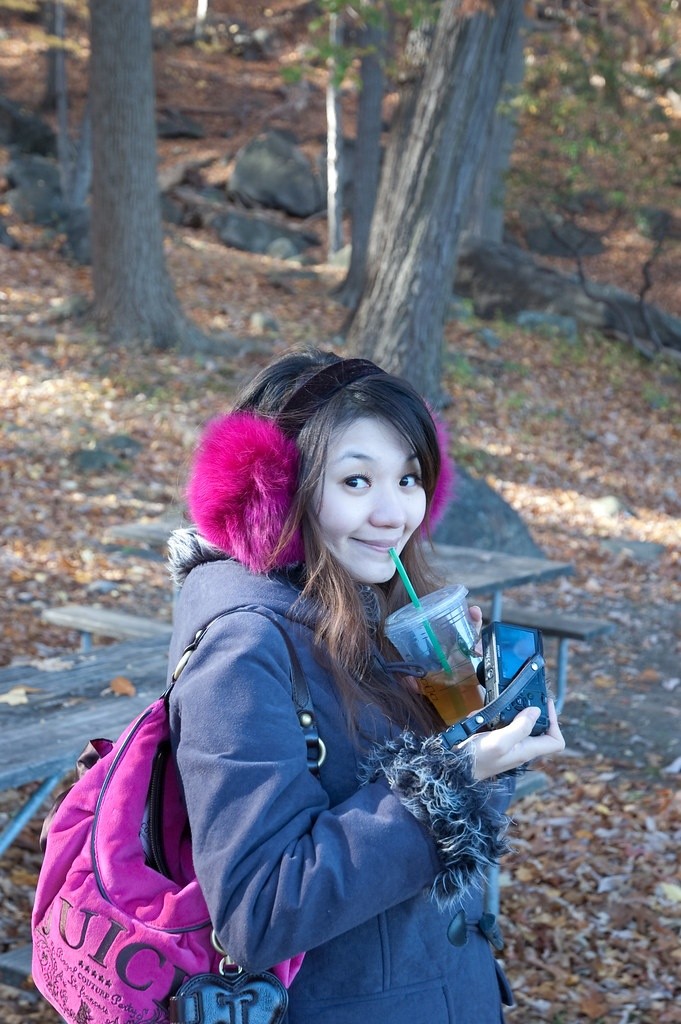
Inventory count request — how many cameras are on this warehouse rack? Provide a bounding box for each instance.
[481,621,551,738]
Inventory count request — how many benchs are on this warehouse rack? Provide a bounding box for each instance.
[43,602,612,725]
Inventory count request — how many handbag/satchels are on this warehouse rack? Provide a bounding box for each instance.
[31,608,327,1024]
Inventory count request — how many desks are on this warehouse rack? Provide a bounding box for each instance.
[0,630,173,855]
[105,499,575,624]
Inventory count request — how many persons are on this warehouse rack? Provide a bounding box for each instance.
[165,350,565,1024]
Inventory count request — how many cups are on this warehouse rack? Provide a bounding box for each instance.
[382,584,485,727]
